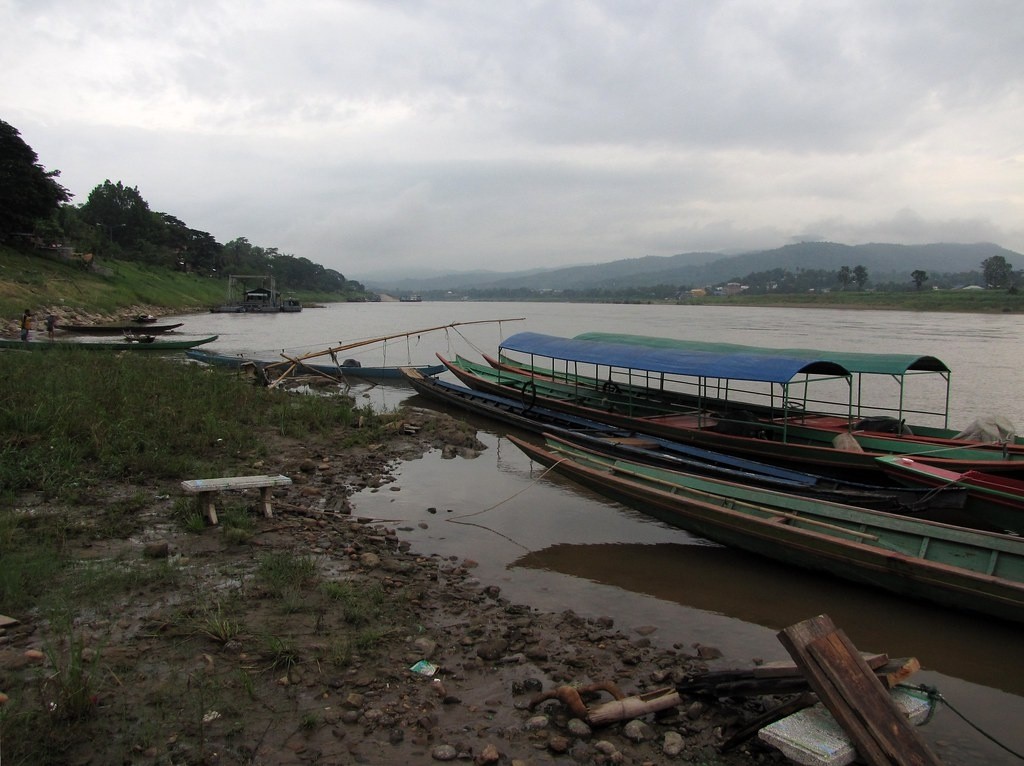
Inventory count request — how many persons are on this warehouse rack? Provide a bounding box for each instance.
[21,309,30,342]
[47,315,55,342]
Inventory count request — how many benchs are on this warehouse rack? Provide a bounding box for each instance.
[786,416,861,428]
[757,679,943,766]
[181,473,292,525]
[601,437,660,449]
[651,414,718,430]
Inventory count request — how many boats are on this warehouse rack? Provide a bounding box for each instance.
[182,349,451,382]
[60,321,185,332]
[210,275,303,314]
[398,329,1024,624]
[0,334,221,350]
[398,294,422,303]
